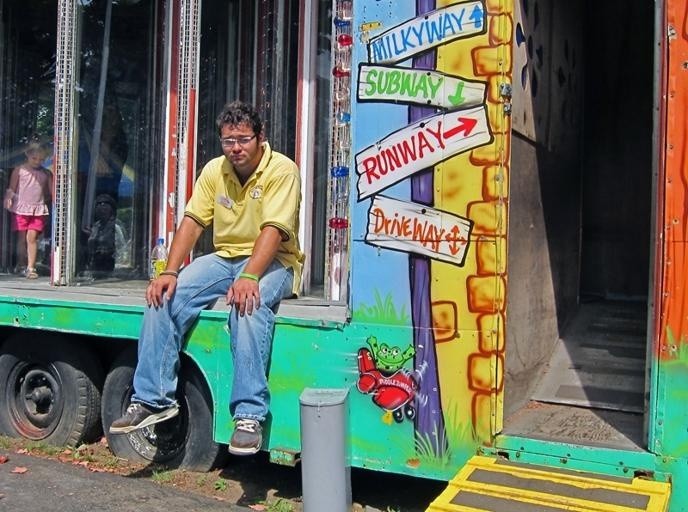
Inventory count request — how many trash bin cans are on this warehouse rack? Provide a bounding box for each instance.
[299,387,352,512]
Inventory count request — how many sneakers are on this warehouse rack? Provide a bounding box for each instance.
[228,417,263,456]
[109,400,180,435]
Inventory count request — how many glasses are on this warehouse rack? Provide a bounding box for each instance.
[222,134,257,146]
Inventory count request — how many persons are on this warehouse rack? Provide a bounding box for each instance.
[86,193,128,267]
[105,100,307,455]
[3,135,54,279]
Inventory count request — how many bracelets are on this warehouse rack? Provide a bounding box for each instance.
[236,272,259,281]
[157,270,178,278]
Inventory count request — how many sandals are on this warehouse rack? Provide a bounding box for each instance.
[14,263,38,279]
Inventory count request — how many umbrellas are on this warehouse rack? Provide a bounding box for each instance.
[0,114,134,196]
